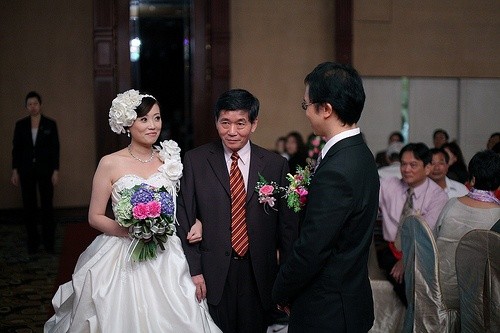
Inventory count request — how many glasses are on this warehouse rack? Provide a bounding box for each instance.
[301,101,317,111]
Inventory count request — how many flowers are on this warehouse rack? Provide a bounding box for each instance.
[112,181,175,263]
[283,164,312,213]
[254,171,280,215]
[307,133,328,160]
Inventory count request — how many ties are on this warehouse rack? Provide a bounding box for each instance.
[394,187,414,252]
[229,152,250,258]
[312,151,322,174]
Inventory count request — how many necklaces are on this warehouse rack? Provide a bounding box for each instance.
[128,142,156,162]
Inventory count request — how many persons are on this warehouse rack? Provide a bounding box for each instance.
[428,146,470,198]
[434,148,500,311]
[11,91,59,247]
[177,88,300,333]
[273,124,500,188]
[379,141,449,309]
[42,88,227,333]
[271,60,380,333]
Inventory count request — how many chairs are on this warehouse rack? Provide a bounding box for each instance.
[455,228,500,333]
[368,241,406,333]
[399,214,461,333]
